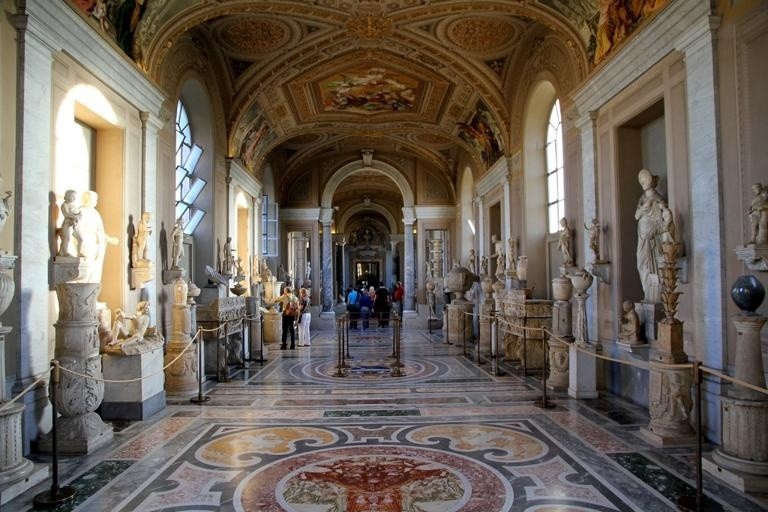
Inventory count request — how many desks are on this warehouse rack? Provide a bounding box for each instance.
[197,296,248,382]
[502,299,552,371]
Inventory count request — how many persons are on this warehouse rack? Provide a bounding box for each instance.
[79,190,314,343]
[658,200,676,243]
[585,219,603,263]
[556,217,575,269]
[634,168,669,299]
[336,280,405,330]
[296,287,313,348]
[52,188,86,259]
[620,297,644,345]
[744,180,768,248]
[467,233,517,278]
[275,286,300,349]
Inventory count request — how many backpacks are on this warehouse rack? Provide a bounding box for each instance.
[283,294,298,316]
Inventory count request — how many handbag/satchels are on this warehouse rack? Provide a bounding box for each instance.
[297,315,302,323]
[347,304,355,311]
